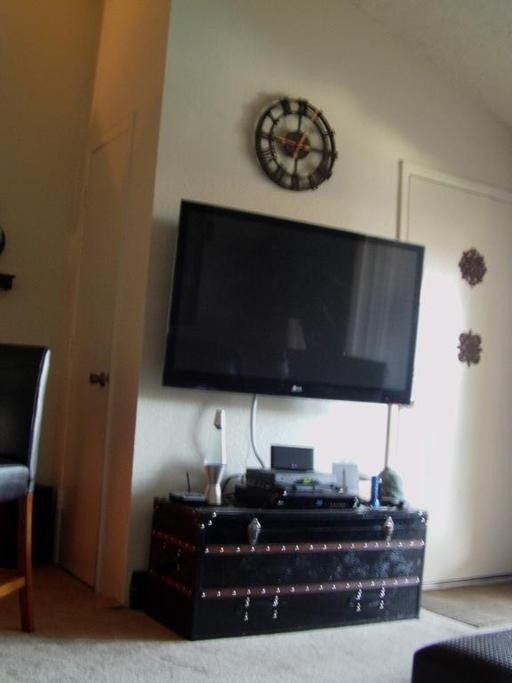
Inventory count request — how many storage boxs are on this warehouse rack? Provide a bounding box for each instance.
[126,491,429,643]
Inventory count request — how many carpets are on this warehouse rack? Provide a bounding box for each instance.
[418,580,511,628]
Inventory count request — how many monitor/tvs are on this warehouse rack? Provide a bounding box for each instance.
[161,198,425,406]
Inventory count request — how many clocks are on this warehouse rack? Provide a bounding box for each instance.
[252,94,339,193]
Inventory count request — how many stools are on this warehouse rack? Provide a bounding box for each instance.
[410,627,512,682]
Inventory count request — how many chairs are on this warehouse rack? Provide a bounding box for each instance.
[0,339,54,637]
